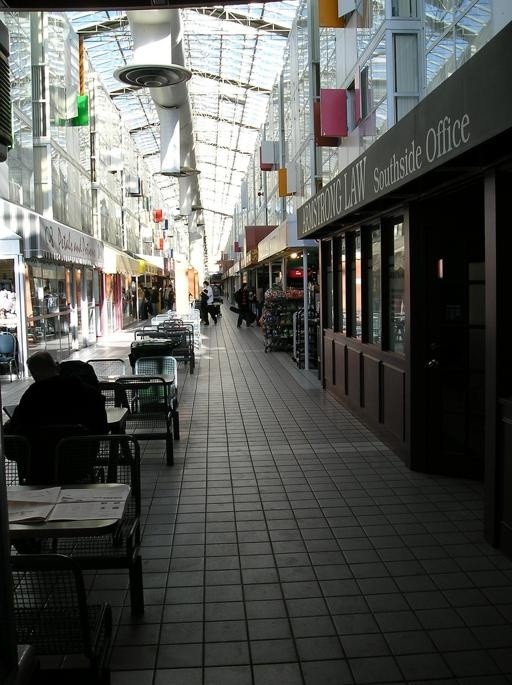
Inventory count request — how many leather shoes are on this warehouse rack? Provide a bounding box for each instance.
[215,318,217,324]
[204,322,209,324]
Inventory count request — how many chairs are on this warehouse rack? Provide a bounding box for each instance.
[86,355,180,465]
[2,406,145,684]
[0,332,19,382]
[128,309,200,373]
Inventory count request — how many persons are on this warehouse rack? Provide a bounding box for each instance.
[27,352,100,484]
[121,286,137,322]
[199,289,209,323]
[201,280,217,325]
[237,282,253,329]
[0,284,68,336]
[213,285,220,296]
[141,284,194,320]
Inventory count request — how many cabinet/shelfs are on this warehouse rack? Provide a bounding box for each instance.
[259,288,320,369]
